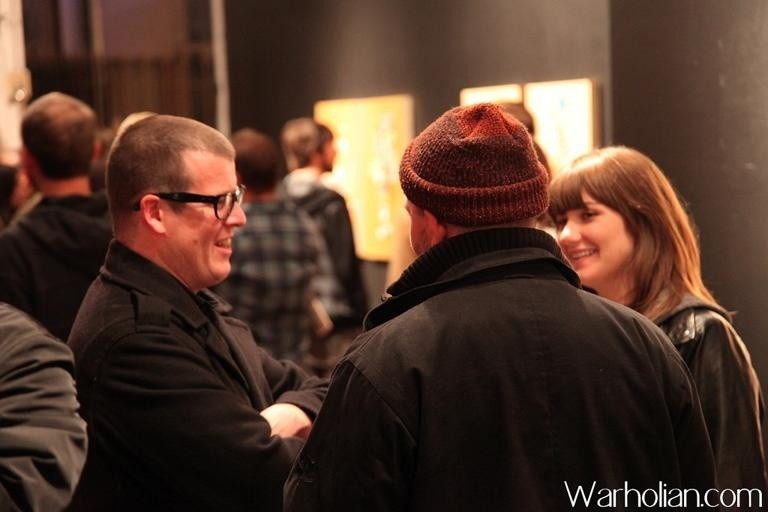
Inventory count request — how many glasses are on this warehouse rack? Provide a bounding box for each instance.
[131,184,247,220]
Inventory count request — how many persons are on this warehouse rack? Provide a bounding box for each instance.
[212,124,345,386]
[542,141,766,511]
[65,109,332,510]
[276,115,369,339]
[277,101,720,512]
[0,159,37,231]
[0,86,114,348]
[1,299,91,511]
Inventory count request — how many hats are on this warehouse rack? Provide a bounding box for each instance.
[399,103,551,226]
